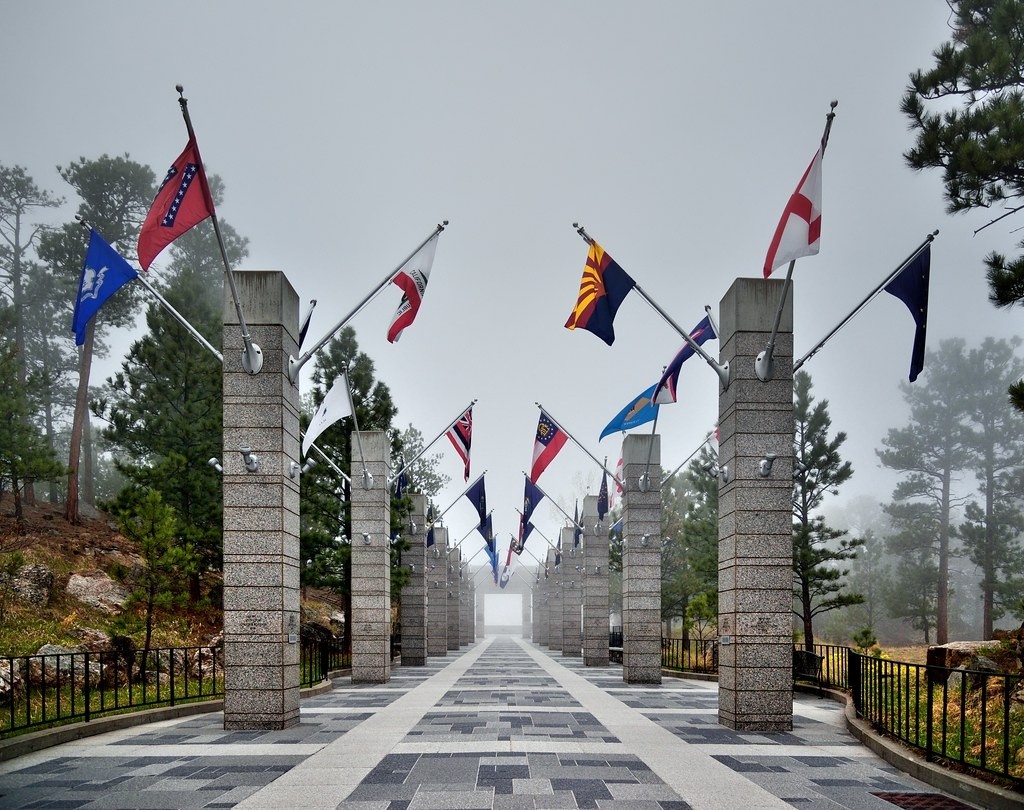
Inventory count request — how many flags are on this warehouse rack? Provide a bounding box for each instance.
[763,140,822,280]
[651,315,718,406]
[597,462,608,520]
[609,447,624,507]
[137,138,214,272]
[386,234,439,344]
[426,507,434,548]
[393,463,406,497]
[302,375,352,456]
[71,230,137,346]
[600,382,659,441]
[445,406,472,482]
[299,311,311,347]
[464,471,583,589]
[709,428,718,455]
[565,241,635,347]
[531,411,568,485]
[884,246,931,382]
